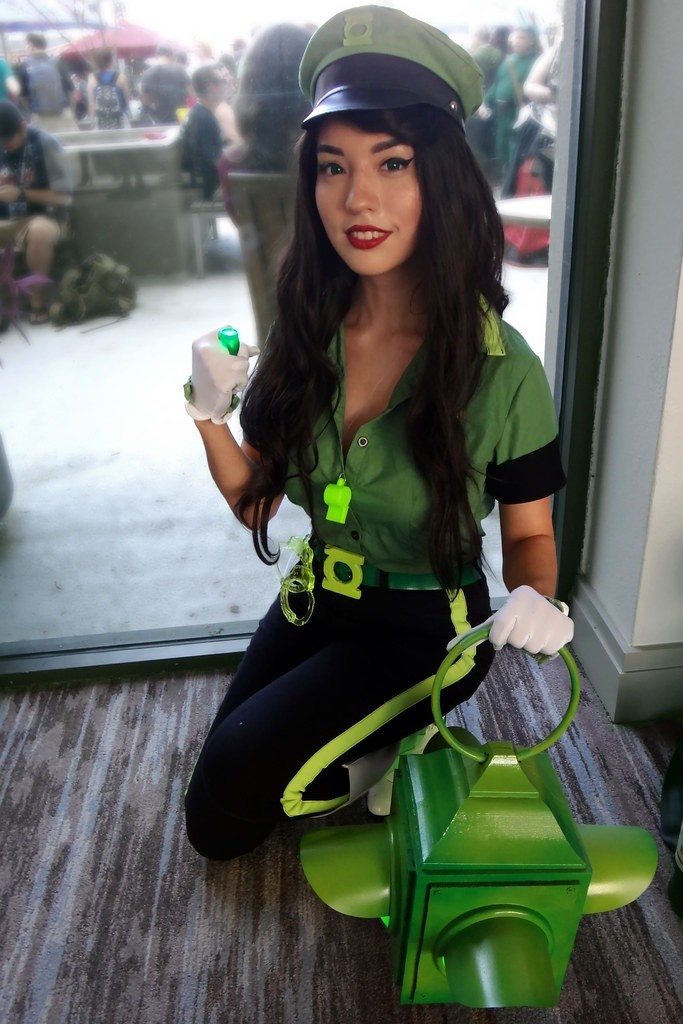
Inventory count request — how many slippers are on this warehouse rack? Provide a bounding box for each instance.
[26,305,50,324]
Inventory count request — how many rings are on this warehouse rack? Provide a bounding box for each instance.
[217,326,240,356]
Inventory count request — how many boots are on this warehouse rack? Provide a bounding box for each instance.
[314,714,442,818]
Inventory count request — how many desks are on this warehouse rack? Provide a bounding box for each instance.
[494,193,552,225]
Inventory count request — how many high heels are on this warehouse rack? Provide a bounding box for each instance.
[75,172,94,187]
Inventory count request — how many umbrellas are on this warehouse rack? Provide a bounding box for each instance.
[0,0,104,59]
[57,19,173,97]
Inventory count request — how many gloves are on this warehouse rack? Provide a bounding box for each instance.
[446,584,573,669]
[183,326,260,426]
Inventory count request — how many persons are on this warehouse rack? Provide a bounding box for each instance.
[184,5,574,861]
[1,19,563,326]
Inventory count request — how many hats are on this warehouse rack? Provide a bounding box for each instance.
[299,7,485,130]
[0,99,24,143]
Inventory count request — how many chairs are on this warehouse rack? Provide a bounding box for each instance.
[227,169,297,353]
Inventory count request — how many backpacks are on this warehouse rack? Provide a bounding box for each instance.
[94,70,123,129]
[19,53,68,118]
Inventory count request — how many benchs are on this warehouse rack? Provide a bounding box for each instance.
[189,187,226,278]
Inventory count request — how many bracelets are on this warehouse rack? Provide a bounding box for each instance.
[15,189,25,204]
